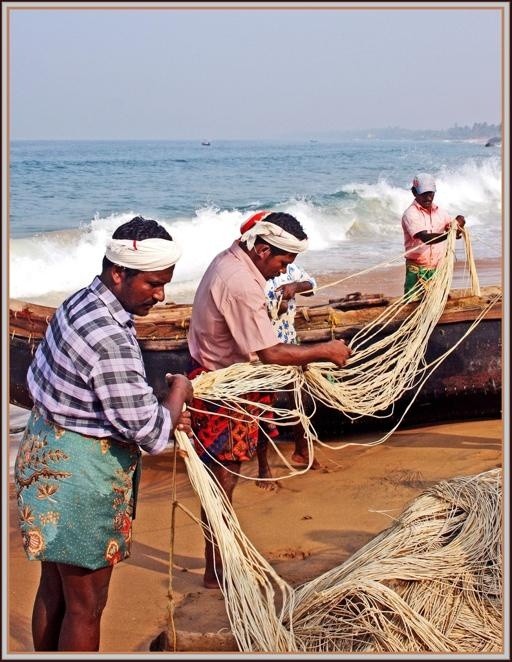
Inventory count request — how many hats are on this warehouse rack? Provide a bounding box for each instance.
[413,173,436,195]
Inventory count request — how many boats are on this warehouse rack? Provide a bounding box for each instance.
[7,288,503,441]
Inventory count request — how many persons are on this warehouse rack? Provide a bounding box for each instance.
[187,213,353,589]
[255,263,321,490]
[402,173,464,304]
[15,218,194,651]
